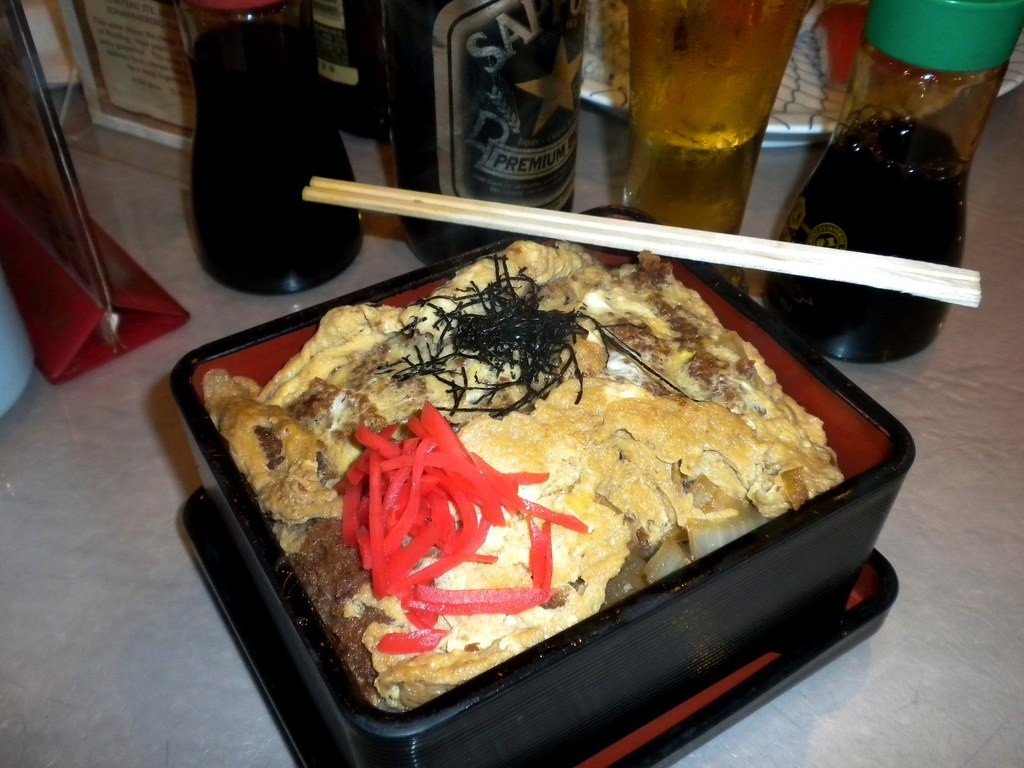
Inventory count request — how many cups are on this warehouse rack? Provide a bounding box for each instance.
[623,0,814,234]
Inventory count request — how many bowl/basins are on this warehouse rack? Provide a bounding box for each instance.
[167,202,898,768]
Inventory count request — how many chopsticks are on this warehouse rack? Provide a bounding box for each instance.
[300,174,983,309]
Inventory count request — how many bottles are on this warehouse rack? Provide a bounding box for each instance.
[177,0,585,295]
[760,0,1024,364]
